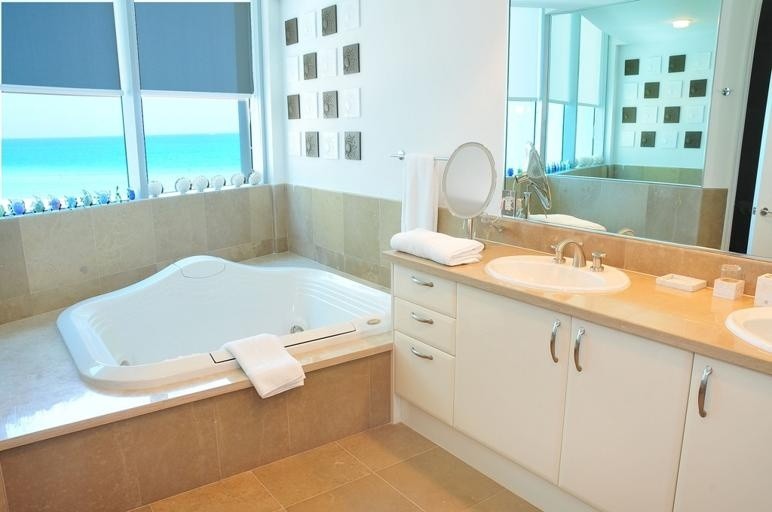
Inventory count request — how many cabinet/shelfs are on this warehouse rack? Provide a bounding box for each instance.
[672,353,772,512]
[390,262,455,453]
[456,284,694,512]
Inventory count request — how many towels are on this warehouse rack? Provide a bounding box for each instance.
[390,227,484,267]
[221,331,307,398]
[400,152,440,234]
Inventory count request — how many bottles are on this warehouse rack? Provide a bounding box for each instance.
[1,186,136,217]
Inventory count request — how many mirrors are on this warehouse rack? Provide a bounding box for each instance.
[513,148,552,218]
[441,141,497,239]
[504,0,771,264]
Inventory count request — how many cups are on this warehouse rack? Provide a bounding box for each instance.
[720,263,741,283]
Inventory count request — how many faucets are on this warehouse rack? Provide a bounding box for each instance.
[617,227,637,236]
[552,238,587,269]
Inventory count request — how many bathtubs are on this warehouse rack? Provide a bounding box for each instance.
[57,255,393,386]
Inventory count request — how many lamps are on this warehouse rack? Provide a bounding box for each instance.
[668,16,694,29]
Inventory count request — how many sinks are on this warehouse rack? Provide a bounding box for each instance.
[482,252,632,294]
[724,306,772,351]
